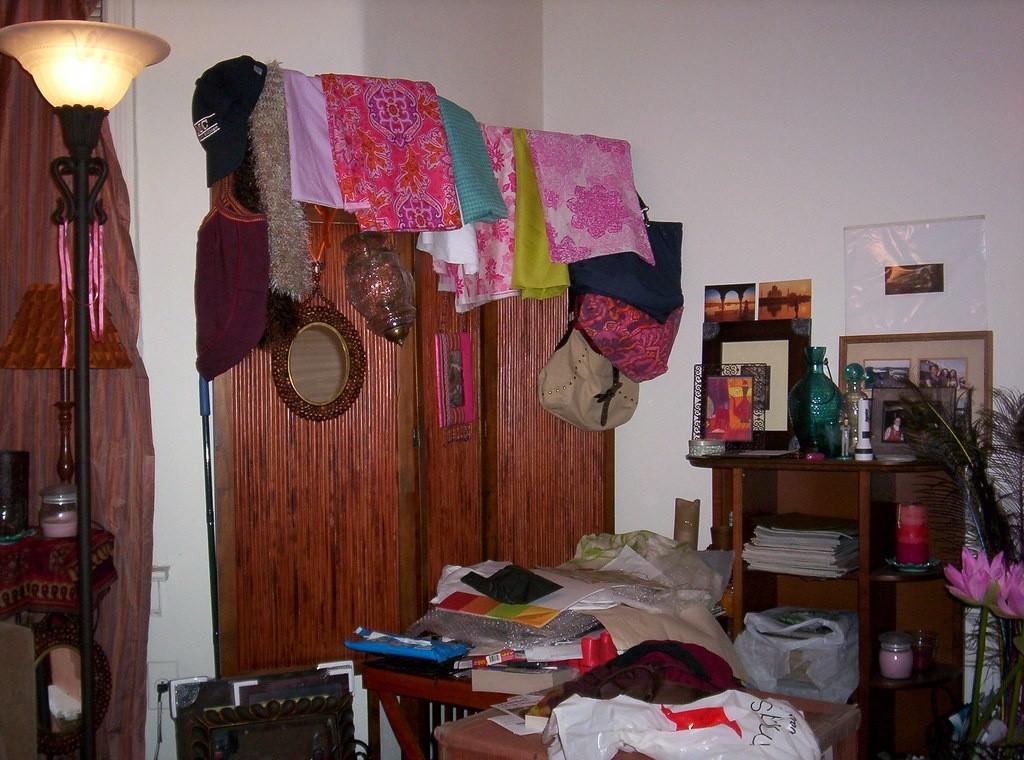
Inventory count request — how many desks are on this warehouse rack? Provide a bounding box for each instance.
[365,668,518,760]
[435,679,864,759]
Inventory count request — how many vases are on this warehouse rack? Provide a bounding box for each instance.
[787,347,843,459]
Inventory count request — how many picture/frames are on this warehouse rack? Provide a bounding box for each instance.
[702,318,812,453]
[838,330,994,457]
[692,363,768,451]
[176,670,349,760]
[871,387,956,455]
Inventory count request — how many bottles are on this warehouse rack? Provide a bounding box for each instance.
[787,347,845,459]
[843,363,868,455]
[38,484,78,538]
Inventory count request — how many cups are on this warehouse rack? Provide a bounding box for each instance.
[909,630,935,671]
[896,501,929,565]
[879,631,916,679]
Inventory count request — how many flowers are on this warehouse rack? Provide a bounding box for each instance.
[944,546,1024,760]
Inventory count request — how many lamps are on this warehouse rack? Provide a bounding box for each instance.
[340,230,419,349]
[0,20,170,760]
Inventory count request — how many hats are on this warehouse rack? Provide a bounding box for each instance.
[192,55,266,187]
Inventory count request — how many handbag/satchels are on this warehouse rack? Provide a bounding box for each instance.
[195,195,270,382]
[541,689,822,760]
[741,604,860,652]
[535,216,684,432]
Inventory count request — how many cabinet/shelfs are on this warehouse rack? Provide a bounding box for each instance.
[686,454,969,760]
[209,123,614,675]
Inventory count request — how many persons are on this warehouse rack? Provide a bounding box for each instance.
[884,418,904,441]
[926,364,959,388]
[448,353,463,396]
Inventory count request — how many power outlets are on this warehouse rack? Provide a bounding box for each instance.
[148,677,169,709]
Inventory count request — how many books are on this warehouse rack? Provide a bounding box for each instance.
[740,525,859,578]
[453,626,606,694]
